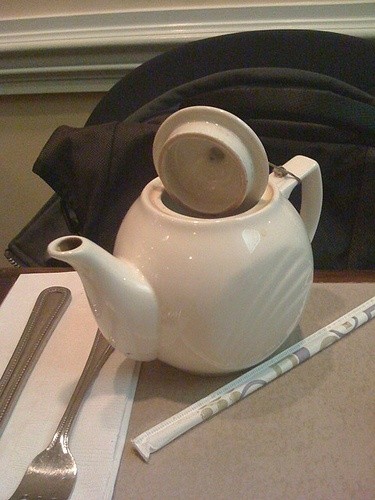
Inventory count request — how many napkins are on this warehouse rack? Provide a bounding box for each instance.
[0,271,142,500]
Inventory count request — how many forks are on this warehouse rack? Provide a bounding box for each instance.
[9,327,116,500]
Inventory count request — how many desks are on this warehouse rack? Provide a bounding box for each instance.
[0,267,375,500]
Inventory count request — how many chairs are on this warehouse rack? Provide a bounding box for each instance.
[11,29,375,269]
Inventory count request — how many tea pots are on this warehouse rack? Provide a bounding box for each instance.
[48,107,323,376]
